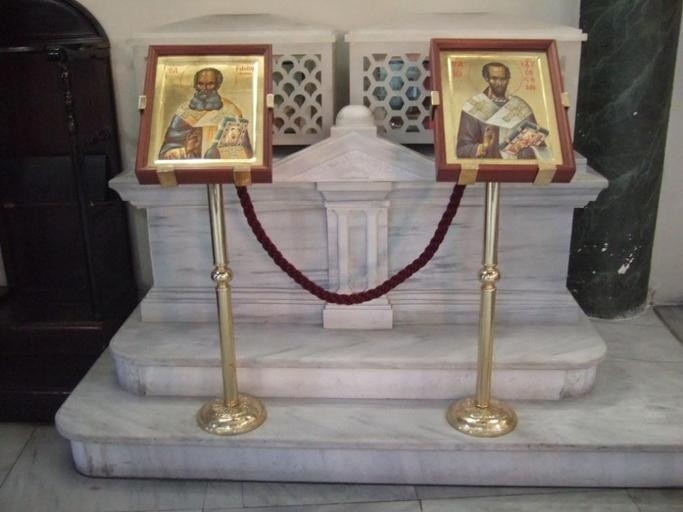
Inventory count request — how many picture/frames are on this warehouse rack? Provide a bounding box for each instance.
[427,38,577,185]
[133,44,273,184]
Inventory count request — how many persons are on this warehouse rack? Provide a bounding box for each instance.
[457,62,550,159]
[158,67,253,159]
